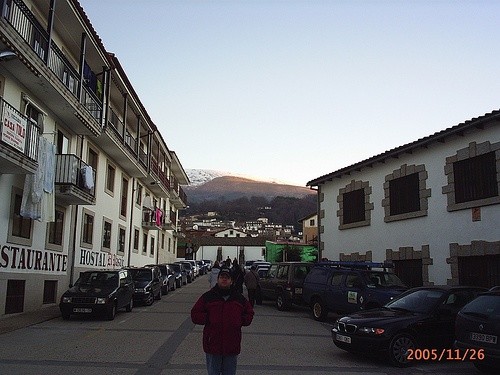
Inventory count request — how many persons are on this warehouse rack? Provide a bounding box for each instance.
[208,256,260,306]
[191,267,254,375]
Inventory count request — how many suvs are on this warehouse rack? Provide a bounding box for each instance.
[119,265,163,306]
[269,261,317,311]
[302,260,412,321]
[60,269,135,321]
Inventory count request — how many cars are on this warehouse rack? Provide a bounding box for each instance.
[143,259,214,295]
[331,285,500,375]
[245,259,271,282]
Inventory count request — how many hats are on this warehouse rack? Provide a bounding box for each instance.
[217,268,233,280]
[251,266,256,269]
